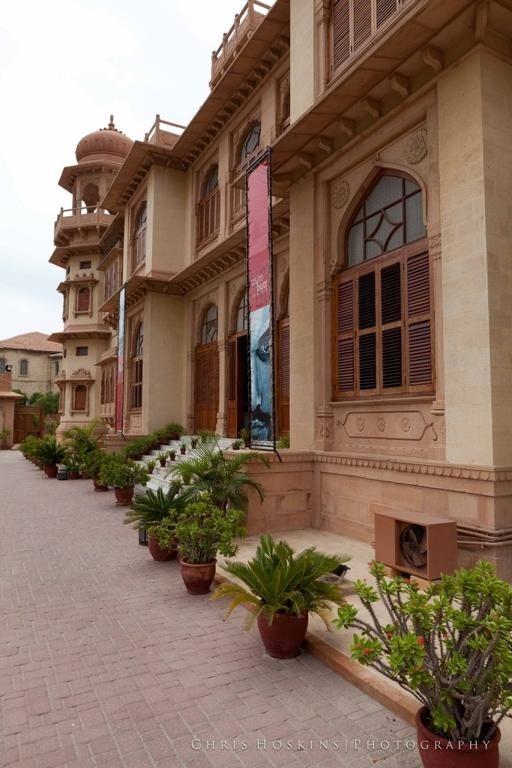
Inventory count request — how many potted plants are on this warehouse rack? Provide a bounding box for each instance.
[17,417,271,600]
[204,530,355,663]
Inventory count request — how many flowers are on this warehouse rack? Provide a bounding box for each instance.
[330,554,511,746]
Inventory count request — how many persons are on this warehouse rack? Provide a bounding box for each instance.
[250,305,274,441]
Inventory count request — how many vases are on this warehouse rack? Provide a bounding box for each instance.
[413,704,502,767]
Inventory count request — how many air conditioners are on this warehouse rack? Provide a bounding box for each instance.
[373,507,460,582]
